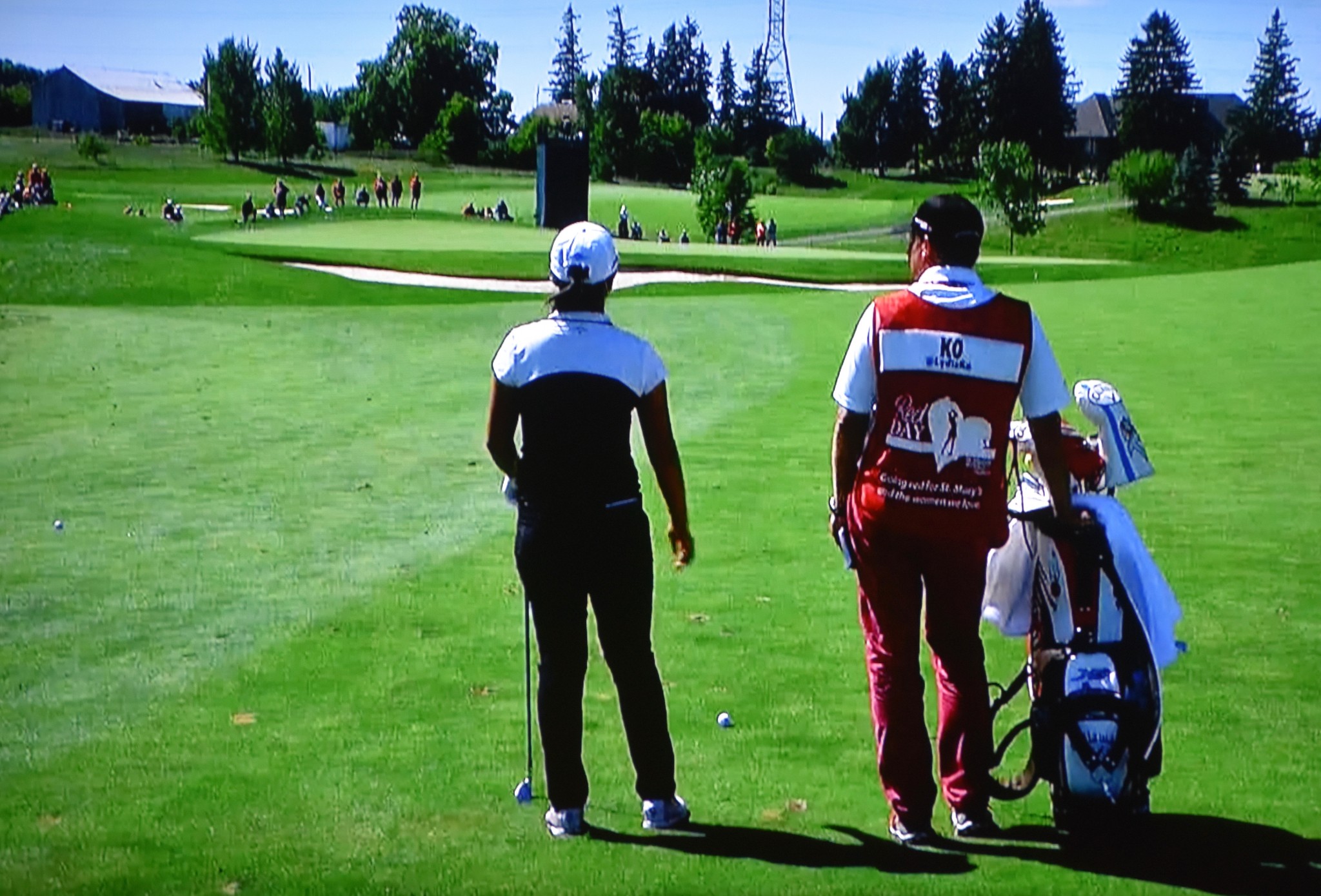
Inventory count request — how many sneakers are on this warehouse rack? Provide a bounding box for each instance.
[642,795,686,827]
[949,807,993,838]
[542,806,585,836]
[888,810,932,844]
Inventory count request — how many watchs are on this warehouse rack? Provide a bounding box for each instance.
[828,495,847,517]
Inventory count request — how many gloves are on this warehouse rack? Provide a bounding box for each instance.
[502,475,518,507]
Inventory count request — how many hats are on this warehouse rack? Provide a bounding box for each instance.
[549,221,618,284]
[913,192,984,243]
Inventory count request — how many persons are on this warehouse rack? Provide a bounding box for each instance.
[487,221,695,839]
[162,171,777,246]
[827,193,1099,845]
[0,160,56,220]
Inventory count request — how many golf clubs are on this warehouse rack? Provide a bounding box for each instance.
[514,591,533,802]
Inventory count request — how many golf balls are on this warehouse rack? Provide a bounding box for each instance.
[715,711,733,728]
[54,520,63,529]
